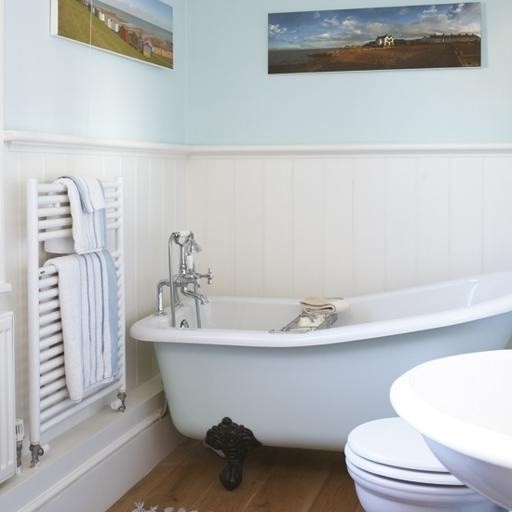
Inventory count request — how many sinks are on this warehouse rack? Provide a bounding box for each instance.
[389,348,511,508]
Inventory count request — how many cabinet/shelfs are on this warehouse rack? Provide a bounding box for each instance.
[0,307,16,487]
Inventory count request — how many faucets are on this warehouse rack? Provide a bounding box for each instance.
[183,286,210,306]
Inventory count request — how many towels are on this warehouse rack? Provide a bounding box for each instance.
[40,251,118,402]
[299,296,350,314]
[44,174,107,255]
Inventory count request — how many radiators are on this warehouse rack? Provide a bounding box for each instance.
[25,176,126,465]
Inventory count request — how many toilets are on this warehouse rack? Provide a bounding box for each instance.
[344,417,500,512]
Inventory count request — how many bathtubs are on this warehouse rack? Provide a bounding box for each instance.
[131,270,512,490]
